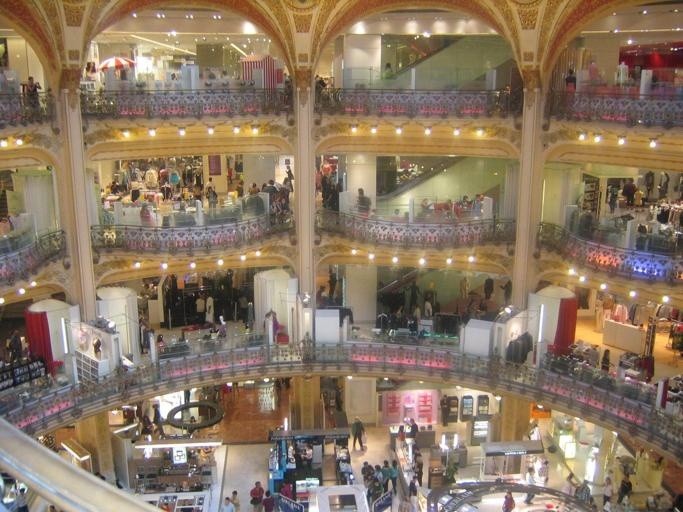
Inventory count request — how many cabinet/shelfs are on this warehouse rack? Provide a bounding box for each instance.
[0,357,47,392]
[74,349,107,386]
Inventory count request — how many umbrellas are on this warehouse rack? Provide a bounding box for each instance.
[98,56,137,80]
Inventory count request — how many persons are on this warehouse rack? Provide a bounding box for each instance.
[280,443,313,500]
[382,275,512,333]
[117,401,162,434]
[196,290,253,329]
[352,416,365,451]
[318,166,485,223]
[589,61,599,81]
[156,335,167,354]
[7,328,23,365]
[17,488,28,512]
[398,418,423,498]
[594,294,612,327]
[284,74,326,106]
[190,416,199,439]
[502,459,634,510]
[361,459,398,507]
[222,481,274,511]
[26,77,42,107]
[566,69,576,92]
[383,62,393,78]
[316,269,343,309]
[570,171,683,259]
[617,62,628,88]
[100,157,294,230]
[590,345,613,372]
